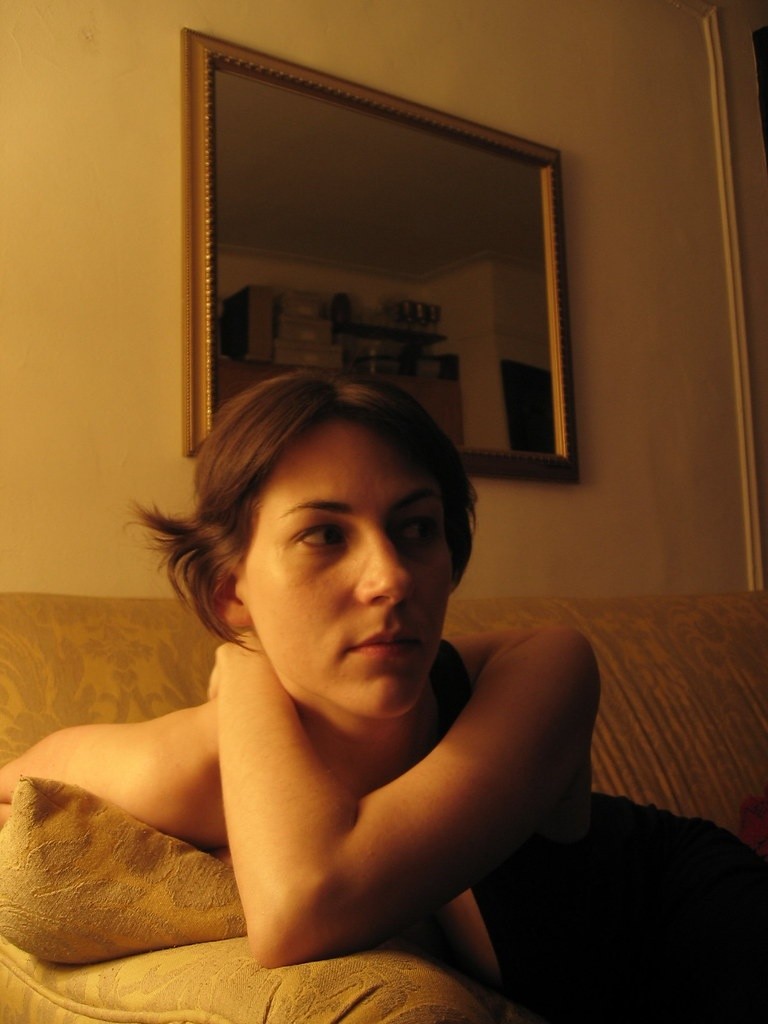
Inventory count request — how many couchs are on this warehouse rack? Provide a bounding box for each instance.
[0,590,768,1021]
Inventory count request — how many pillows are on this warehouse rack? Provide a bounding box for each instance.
[0,780,247,967]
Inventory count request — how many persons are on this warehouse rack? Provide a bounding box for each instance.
[0,369,767,1024]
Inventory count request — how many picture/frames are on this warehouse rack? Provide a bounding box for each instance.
[178,28,582,489]
